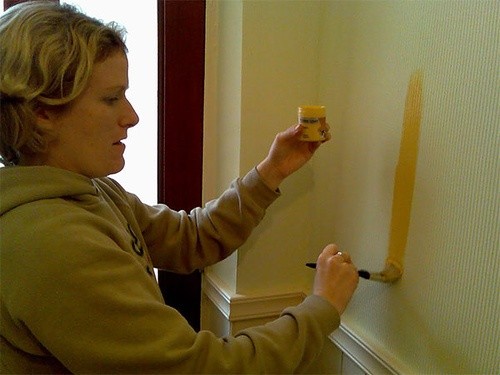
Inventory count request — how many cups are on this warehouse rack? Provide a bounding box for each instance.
[297,105,327,142]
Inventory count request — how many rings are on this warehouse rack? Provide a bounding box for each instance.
[335,251,349,263]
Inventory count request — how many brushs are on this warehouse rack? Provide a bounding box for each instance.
[305,259,402,283]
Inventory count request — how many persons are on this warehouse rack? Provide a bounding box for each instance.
[0,0,360,375]
